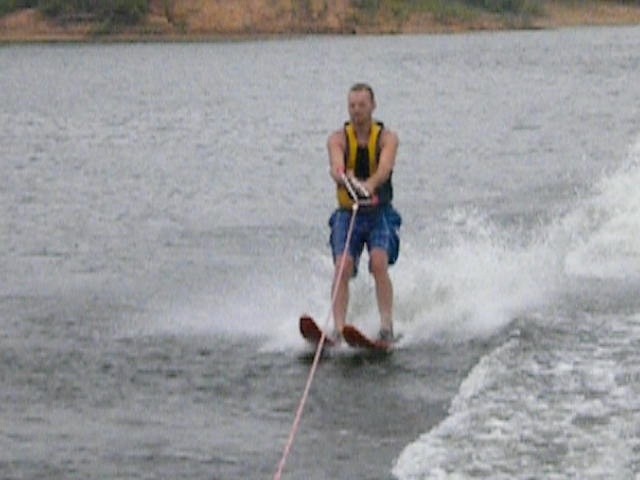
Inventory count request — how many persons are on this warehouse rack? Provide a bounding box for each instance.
[316,81,403,350]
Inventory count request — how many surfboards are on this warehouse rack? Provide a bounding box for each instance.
[342,324,387,354]
[298,314,335,348]
